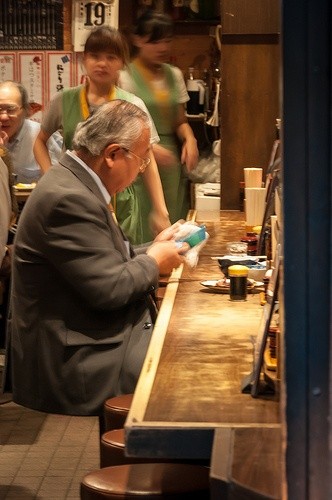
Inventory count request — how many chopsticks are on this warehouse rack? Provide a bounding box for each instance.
[210,257,267,261]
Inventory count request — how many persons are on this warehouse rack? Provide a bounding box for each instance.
[4,100,191,418]
[0,81,64,324]
[32,26,173,251]
[122,10,200,226]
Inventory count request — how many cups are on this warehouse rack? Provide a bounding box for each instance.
[245,188,267,226]
[244,168,262,188]
[228,265,249,300]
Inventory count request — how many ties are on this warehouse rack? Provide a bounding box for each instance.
[108,201,119,226]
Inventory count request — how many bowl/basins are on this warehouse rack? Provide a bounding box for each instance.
[245,265,267,281]
[220,266,228,278]
[217,259,256,266]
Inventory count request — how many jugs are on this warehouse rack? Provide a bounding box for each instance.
[186,80,205,115]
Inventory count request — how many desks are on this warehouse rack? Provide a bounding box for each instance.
[124,209,285,462]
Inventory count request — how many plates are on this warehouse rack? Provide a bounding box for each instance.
[12,184,36,190]
[200,279,264,293]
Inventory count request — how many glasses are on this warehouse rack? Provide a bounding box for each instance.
[122,148,150,173]
[0,106,24,117]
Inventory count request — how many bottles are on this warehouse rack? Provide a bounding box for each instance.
[262,304,277,369]
[240,181,245,212]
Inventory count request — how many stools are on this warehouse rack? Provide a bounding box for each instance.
[80,393,208,500]
